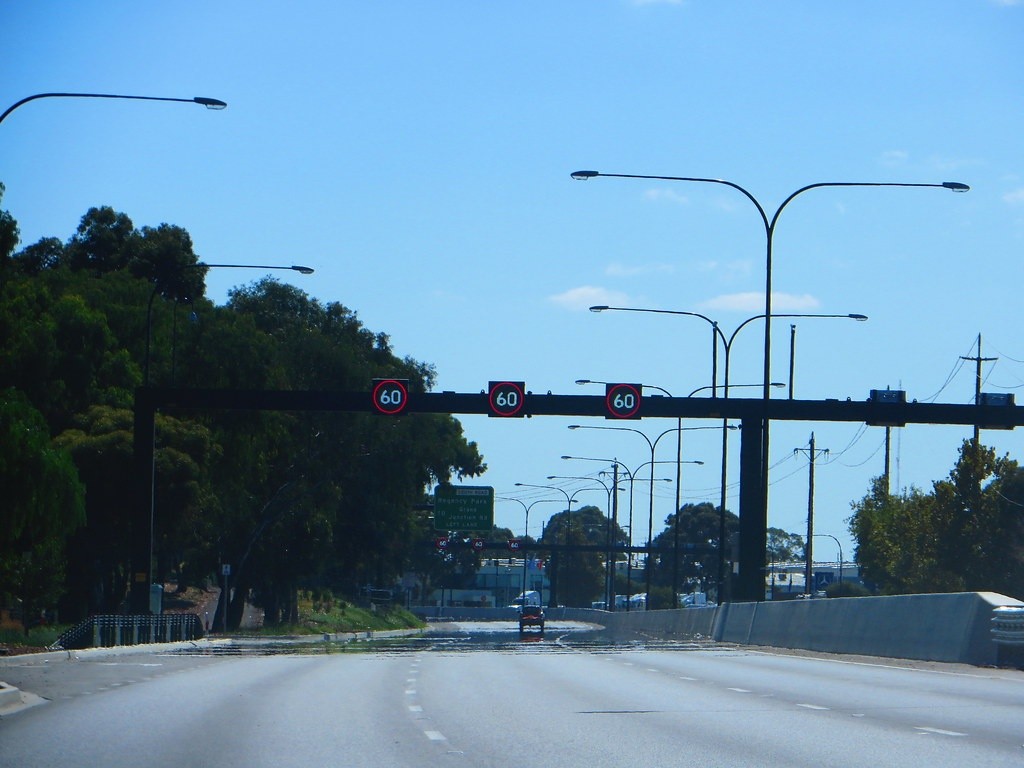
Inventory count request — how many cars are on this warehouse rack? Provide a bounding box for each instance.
[591,592,717,607]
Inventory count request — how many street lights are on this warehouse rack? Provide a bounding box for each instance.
[514,483,626,608]
[494,496,578,606]
[561,454,705,611]
[569,170,972,593]
[134,265,315,616]
[569,425,739,611]
[588,305,869,604]
[575,379,788,607]
[546,474,672,613]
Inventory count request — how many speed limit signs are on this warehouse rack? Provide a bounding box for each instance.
[373,380,408,415]
[489,382,525,417]
[606,382,641,419]
[510,539,520,551]
[437,537,449,550]
[472,539,484,551]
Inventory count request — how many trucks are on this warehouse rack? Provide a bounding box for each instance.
[507,590,541,614]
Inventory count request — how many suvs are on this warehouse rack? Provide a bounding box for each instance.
[517,604,546,634]
